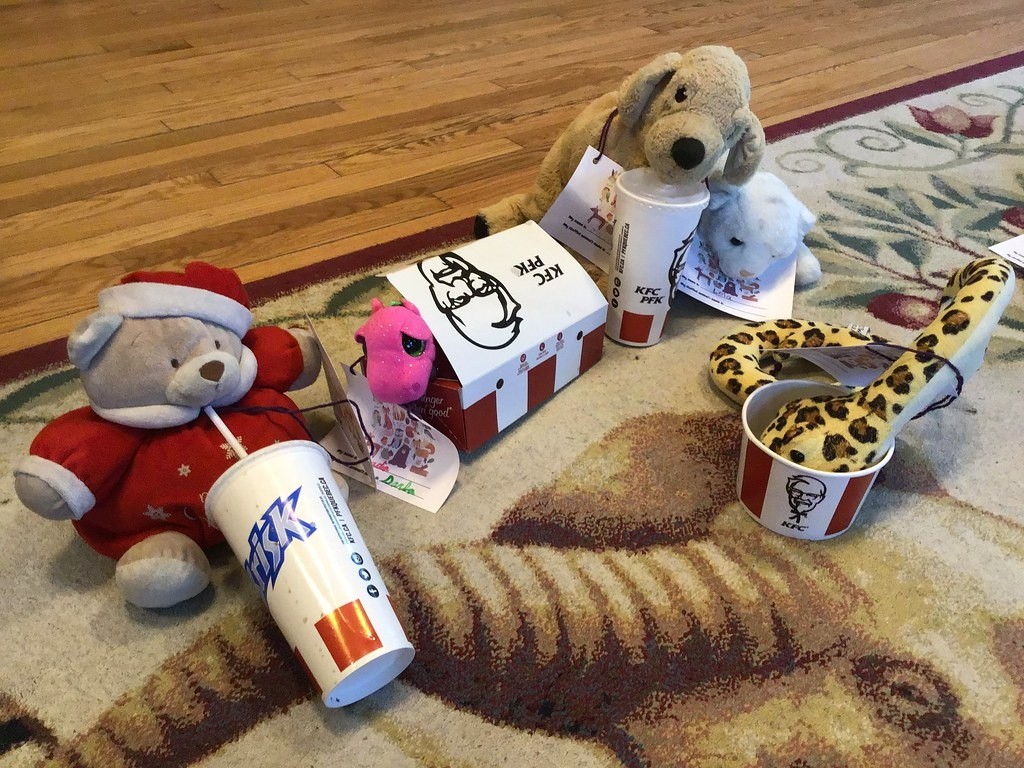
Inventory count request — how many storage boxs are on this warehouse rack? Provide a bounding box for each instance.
[374,218,610,454]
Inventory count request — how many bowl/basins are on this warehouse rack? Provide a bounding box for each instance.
[735,380,896,542]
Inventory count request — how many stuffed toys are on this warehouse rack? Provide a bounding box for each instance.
[700,169,820,285]
[354,297,436,404]
[13,262,348,609]
[473,45,766,238]
[708,254,1017,473]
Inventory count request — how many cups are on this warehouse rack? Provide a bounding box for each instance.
[205,439,417,709]
[605,168,710,348]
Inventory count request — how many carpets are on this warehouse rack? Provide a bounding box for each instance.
[0,50,1024,768]
